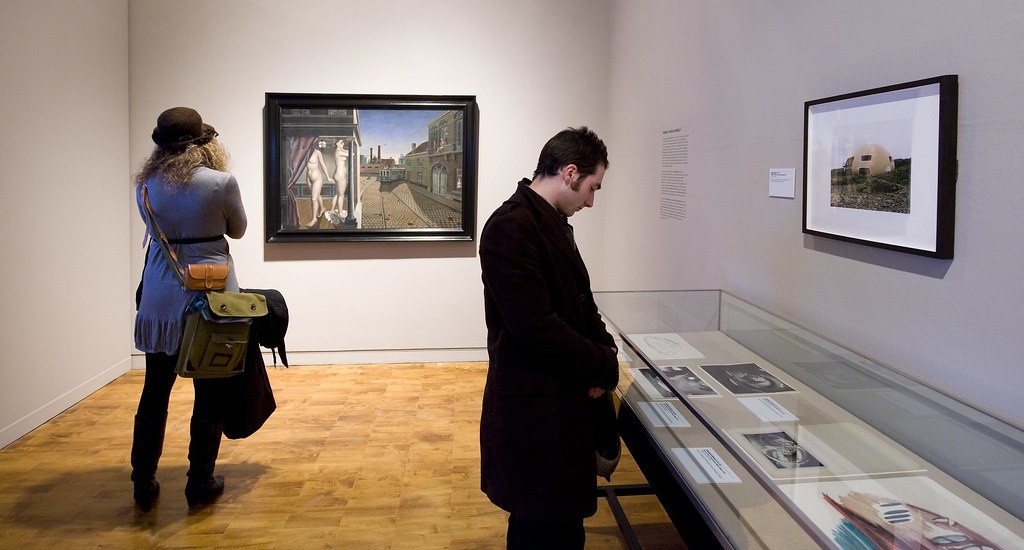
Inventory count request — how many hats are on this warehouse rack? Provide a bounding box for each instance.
[152,107,219,151]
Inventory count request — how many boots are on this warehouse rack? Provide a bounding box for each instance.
[130,411,168,512]
[184,416,224,509]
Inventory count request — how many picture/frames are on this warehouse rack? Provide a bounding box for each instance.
[261,90,479,245]
[796,74,959,259]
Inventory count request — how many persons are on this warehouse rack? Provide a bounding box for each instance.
[479,127,619,550]
[131,107,247,510]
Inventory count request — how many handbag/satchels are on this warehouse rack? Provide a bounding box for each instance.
[174,289,268,380]
[184,264,228,290]
